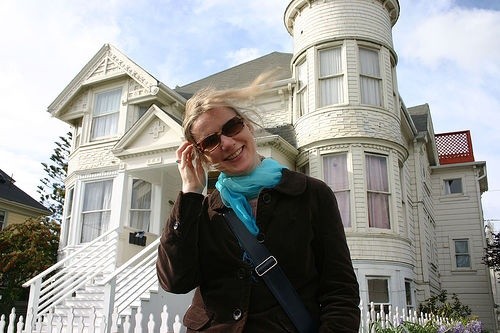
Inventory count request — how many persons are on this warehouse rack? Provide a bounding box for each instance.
[155,87,361,333]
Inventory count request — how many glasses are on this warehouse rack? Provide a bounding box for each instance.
[193,112,246,155]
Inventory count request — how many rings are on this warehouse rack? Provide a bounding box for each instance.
[175,160,181,164]
